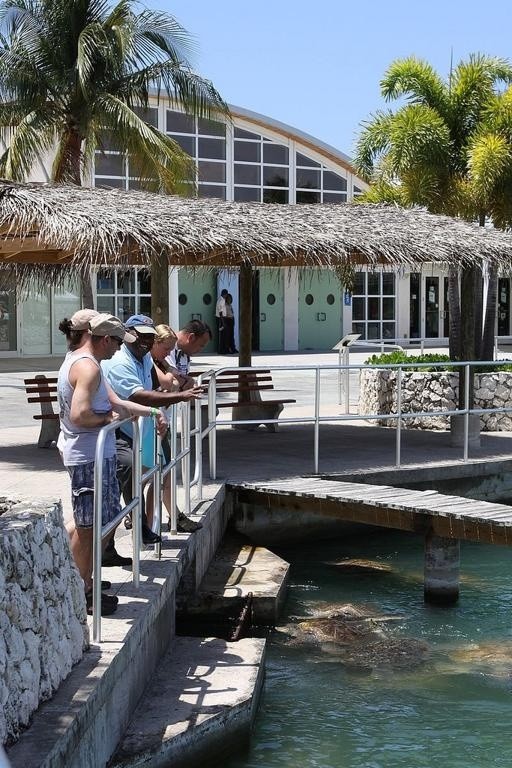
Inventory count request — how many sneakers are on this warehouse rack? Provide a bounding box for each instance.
[167,512,202,533]
[90,578,111,592]
[142,515,161,544]
[101,539,132,567]
[85,591,118,616]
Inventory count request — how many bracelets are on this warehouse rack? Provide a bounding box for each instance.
[149,406,157,418]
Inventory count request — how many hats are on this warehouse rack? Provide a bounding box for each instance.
[68,309,101,331]
[125,314,160,337]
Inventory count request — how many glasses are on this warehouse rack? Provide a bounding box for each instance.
[111,336,124,346]
[87,312,137,344]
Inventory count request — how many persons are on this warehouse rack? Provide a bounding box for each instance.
[215,289,229,355]
[225,294,239,354]
[61,311,137,616]
[160,319,214,532]
[58,307,169,567]
[146,323,195,536]
[100,312,205,544]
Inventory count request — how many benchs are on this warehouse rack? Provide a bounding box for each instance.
[24,368,297,448]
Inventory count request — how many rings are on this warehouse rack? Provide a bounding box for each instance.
[165,428,168,431]
